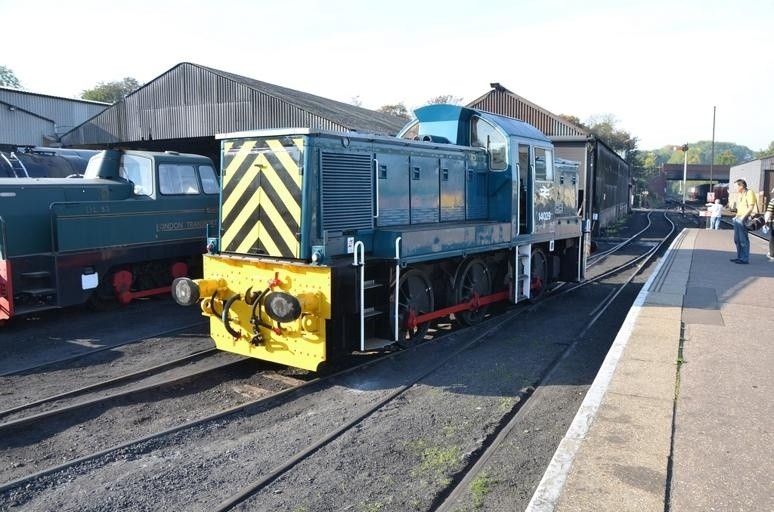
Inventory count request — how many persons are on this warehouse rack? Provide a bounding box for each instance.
[708,199,723,230]
[730,179,756,264]
[765,188,774,259]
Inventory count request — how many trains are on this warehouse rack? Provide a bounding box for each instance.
[172,103,598,375]
[0,145,219,320]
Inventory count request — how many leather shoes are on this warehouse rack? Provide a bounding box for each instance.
[729,258,748,264]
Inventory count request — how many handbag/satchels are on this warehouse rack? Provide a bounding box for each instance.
[744,214,765,231]
